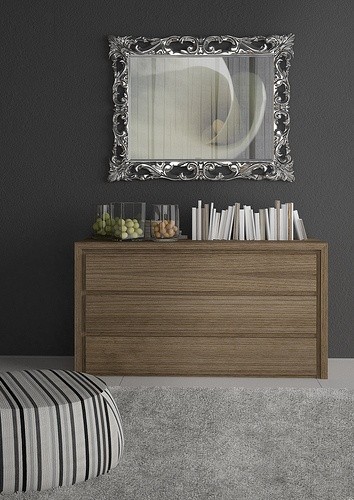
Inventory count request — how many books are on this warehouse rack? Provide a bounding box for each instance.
[191,200,307,240]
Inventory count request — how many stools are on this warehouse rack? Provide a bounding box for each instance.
[0,367,124,495]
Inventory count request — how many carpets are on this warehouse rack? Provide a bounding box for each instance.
[0,387,354,500]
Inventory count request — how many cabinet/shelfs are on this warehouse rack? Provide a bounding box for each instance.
[74,238,329,380]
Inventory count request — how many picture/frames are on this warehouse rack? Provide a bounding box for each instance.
[107,32,297,184]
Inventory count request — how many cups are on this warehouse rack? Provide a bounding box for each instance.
[93,202,181,244]
